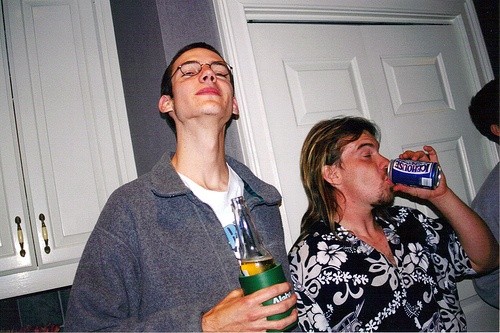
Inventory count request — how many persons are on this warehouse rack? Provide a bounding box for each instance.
[64,43,299,333]
[287,118,500,333]
[468,79,500,309]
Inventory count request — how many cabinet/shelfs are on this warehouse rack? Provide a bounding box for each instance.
[0,0,139,300]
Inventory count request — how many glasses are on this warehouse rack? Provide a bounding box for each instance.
[167,60,233,84]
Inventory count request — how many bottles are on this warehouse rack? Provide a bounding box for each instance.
[230,196,299,333]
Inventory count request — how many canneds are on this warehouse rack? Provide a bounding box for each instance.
[386,158,442,190]
